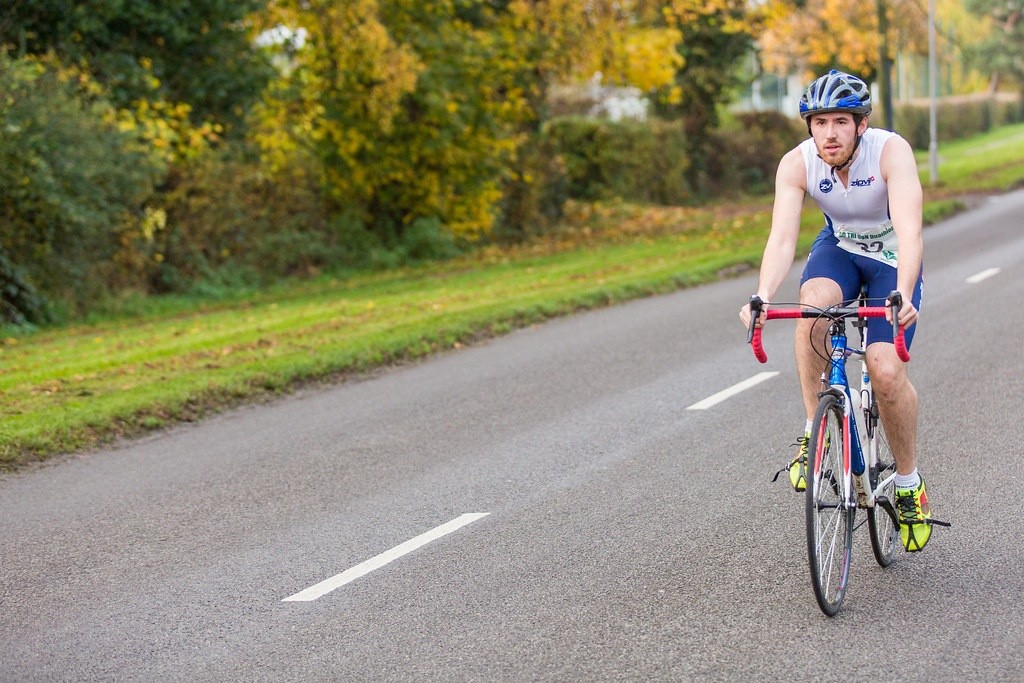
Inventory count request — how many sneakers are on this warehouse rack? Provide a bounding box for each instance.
[895,471,952,552]
[770,428,831,492]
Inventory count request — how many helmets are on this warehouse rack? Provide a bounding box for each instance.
[798,68,873,119]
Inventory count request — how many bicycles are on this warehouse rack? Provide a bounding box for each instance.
[747,282,900,617]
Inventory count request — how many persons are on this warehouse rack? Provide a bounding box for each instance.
[738,68,952,552]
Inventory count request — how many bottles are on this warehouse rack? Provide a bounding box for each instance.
[847,387,868,448]
